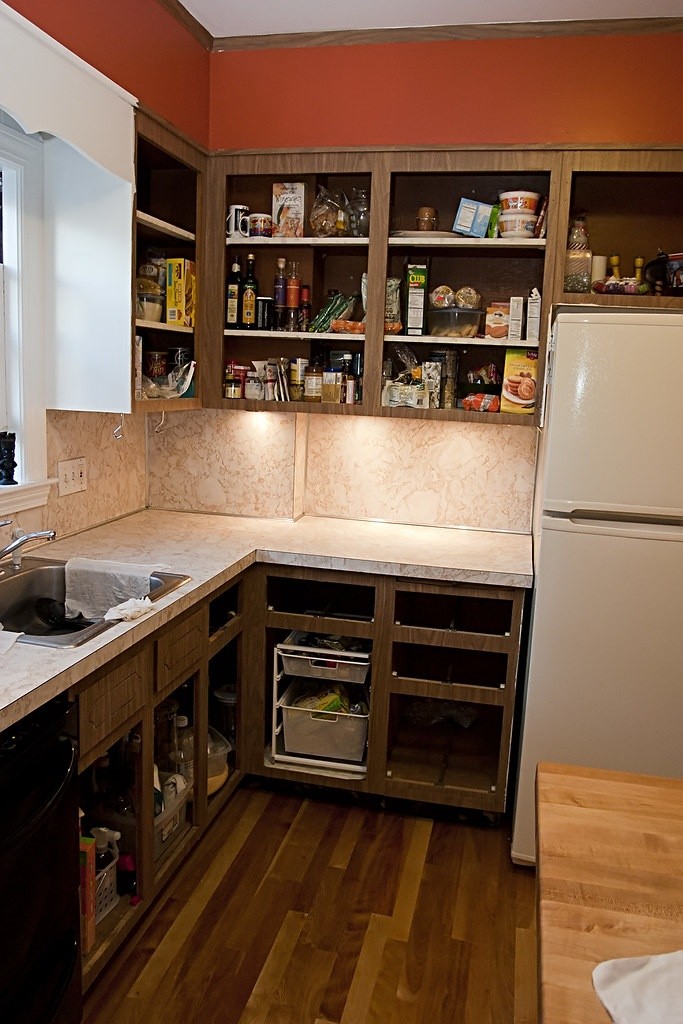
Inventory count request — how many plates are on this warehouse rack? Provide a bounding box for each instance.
[390,230,465,239]
[503,378,535,404]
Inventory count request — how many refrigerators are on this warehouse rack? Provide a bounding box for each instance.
[509,312,683,867]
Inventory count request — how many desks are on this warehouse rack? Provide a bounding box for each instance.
[532,762,683,1024]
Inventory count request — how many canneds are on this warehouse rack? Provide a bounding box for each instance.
[142,347,191,380]
[224,355,345,404]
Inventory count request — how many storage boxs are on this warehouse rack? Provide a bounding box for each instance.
[279,678,370,762]
[88,768,194,858]
[96,847,121,927]
[176,722,232,779]
[499,347,538,414]
[403,255,430,337]
[277,630,372,684]
[452,197,494,238]
[271,182,309,237]
[165,258,197,328]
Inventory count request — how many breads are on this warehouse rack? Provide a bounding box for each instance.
[310,207,346,237]
[485,324,510,338]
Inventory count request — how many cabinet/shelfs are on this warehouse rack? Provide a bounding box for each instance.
[255,563,525,812]
[67,563,247,997]
[206,142,683,425]
[127,97,199,413]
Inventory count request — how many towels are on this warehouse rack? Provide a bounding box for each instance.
[591,949,683,1024]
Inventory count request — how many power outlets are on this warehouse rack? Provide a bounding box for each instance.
[56,457,87,496]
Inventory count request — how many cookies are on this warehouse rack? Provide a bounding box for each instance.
[432,285,482,310]
[505,371,537,401]
[430,324,480,338]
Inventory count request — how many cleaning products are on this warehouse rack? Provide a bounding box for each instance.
[89,827,121,877]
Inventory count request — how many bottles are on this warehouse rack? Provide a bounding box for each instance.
[324,288,338,305]
[225,350,363,405]
[226,255,242,329]
[172,716,194,762]
[286,261,300,332]
[239,254,257,330]
[298,285,311,332]
[349,187,370,237]
[270,258,287,331]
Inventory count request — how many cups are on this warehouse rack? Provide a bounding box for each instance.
[238,213,272,238]
[144,347,192,379]
[255,296,274,329]
[225,205,249,238]
[138,294,164,322]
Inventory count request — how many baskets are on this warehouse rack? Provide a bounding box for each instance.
[94,848,120,926]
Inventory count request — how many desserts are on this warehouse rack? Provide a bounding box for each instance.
[185,271,194,316]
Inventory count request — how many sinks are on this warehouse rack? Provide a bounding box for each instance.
[0,556,194,650]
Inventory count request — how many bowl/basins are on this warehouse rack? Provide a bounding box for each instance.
[425,309,484,337]
[498,215,539,238]
[498,190,540,214]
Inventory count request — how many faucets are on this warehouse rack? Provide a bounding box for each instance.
[0,520,56,561]
